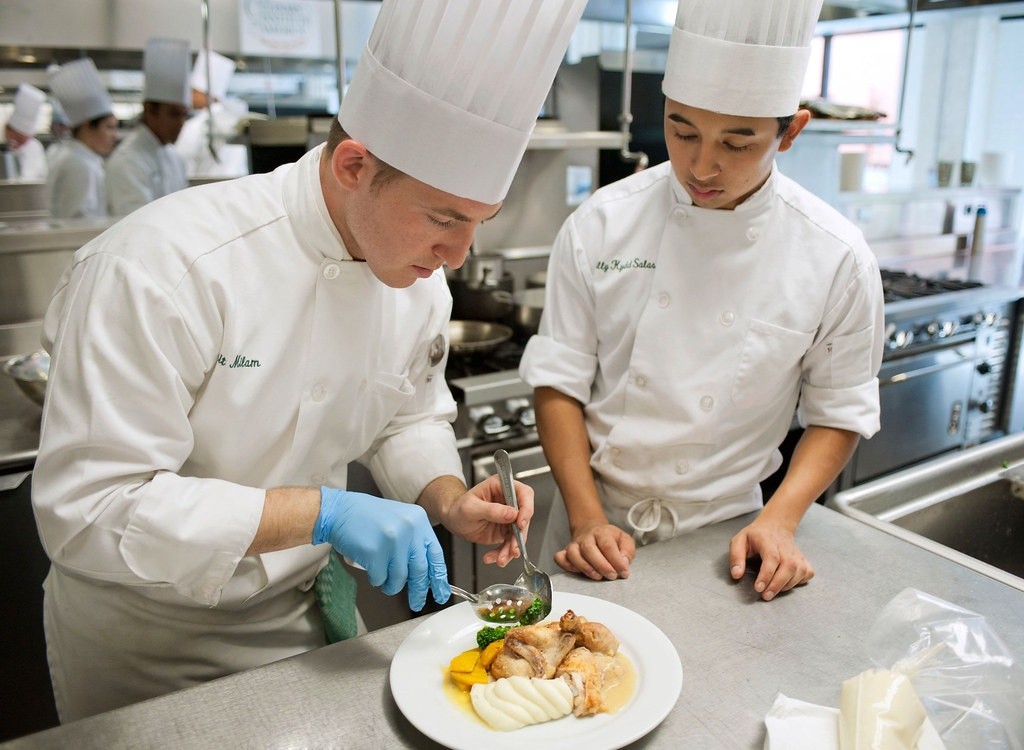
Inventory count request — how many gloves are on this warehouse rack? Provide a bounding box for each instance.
[310,482,453,613]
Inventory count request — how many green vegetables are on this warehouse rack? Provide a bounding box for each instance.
[476,597,542,650]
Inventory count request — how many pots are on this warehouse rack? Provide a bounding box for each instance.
[443,318,514,359]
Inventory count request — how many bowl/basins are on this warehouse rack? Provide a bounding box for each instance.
[3,350,51,411]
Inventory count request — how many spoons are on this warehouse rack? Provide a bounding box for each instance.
[491,450,552,626]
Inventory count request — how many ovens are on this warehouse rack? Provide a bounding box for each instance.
[454,456,607,599]
[846,343,976,481]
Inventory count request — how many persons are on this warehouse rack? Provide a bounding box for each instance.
[31,0,589,723]
[4,81,48,178]
[105,33,191,216]
[47,57,118,218]
[50,97,70,141]
[517,0,886,602]
[174,49,249,179]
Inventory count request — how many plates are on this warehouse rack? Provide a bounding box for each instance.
[389,589,683,750]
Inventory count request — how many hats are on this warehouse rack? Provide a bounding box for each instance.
[335,0,588,205]
[6,83,47,136]
[193,47,236,103]
[50,58,112,128]
[139,37,193,108]
[661,0,823,118]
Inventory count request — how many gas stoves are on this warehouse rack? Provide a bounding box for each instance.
[847,263,1006,361]
[439,284,572,452]
